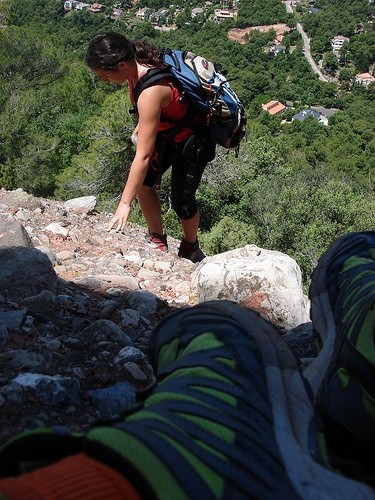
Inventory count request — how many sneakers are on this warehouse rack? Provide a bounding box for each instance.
[148,233,168,254]
[0,300,375,500]
[178,240,206,264]
[298,230,375,483]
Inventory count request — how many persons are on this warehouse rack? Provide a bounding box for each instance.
[84,31,248,263]
[1,229,375,499]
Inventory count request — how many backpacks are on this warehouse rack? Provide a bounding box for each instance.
[133,48,247,149]
